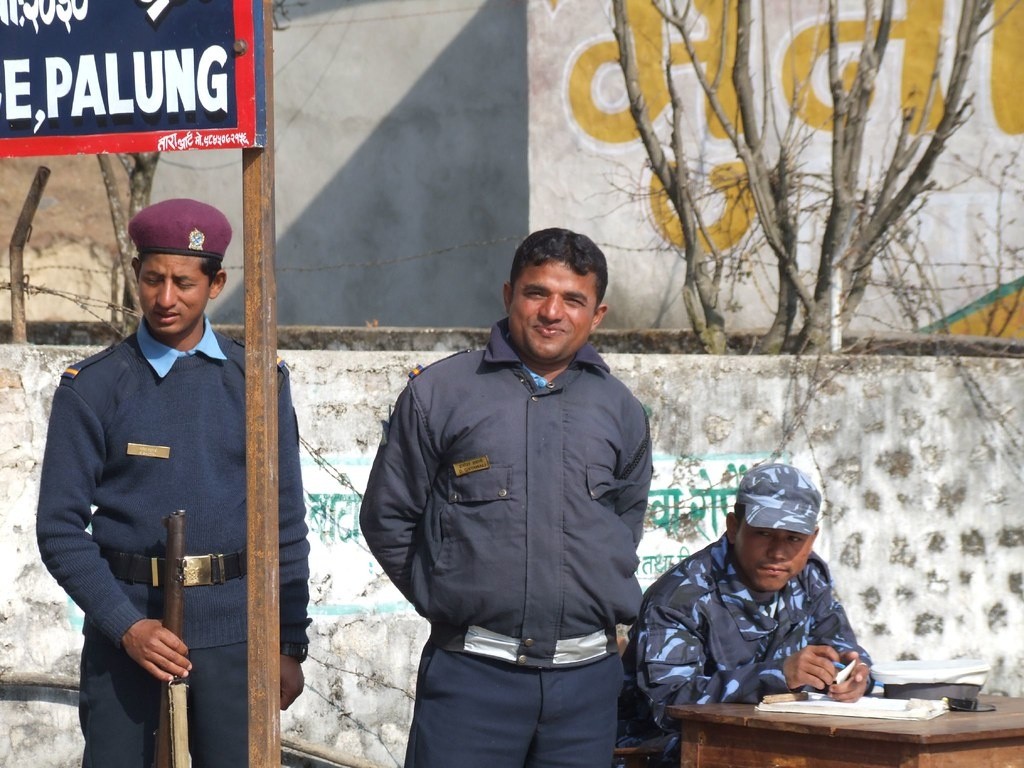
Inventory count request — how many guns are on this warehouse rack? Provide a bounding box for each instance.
[152,507,195,768]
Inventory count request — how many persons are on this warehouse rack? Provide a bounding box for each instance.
[359,228,654,768]
[36,197,313,768]
[611,465,875,768]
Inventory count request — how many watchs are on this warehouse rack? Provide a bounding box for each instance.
[280,643,308,663]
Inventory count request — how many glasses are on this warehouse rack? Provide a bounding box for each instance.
[946,696,997,712]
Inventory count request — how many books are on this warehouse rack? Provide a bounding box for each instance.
[755,691,949,723]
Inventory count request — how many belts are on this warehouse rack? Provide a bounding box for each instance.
[100,544,248,587]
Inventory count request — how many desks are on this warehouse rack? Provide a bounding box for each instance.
[666,694,1024,768]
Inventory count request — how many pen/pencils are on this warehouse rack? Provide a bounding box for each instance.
[831,661,847,669]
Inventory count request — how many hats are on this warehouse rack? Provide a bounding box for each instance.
[736,463,822,535]
[128,198,232,261]
